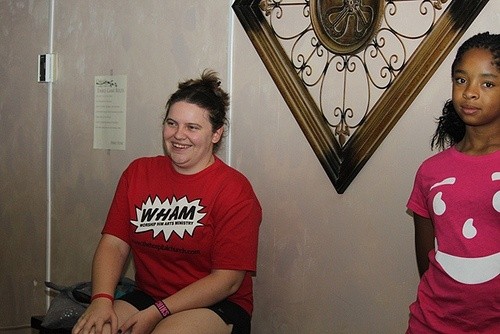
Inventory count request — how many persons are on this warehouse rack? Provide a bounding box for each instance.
[402,30,500,334]
[70,67,265,334]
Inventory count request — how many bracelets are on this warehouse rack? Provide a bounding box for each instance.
[154,300,172,319]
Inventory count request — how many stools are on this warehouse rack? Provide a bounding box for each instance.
[32,311,88,334]
